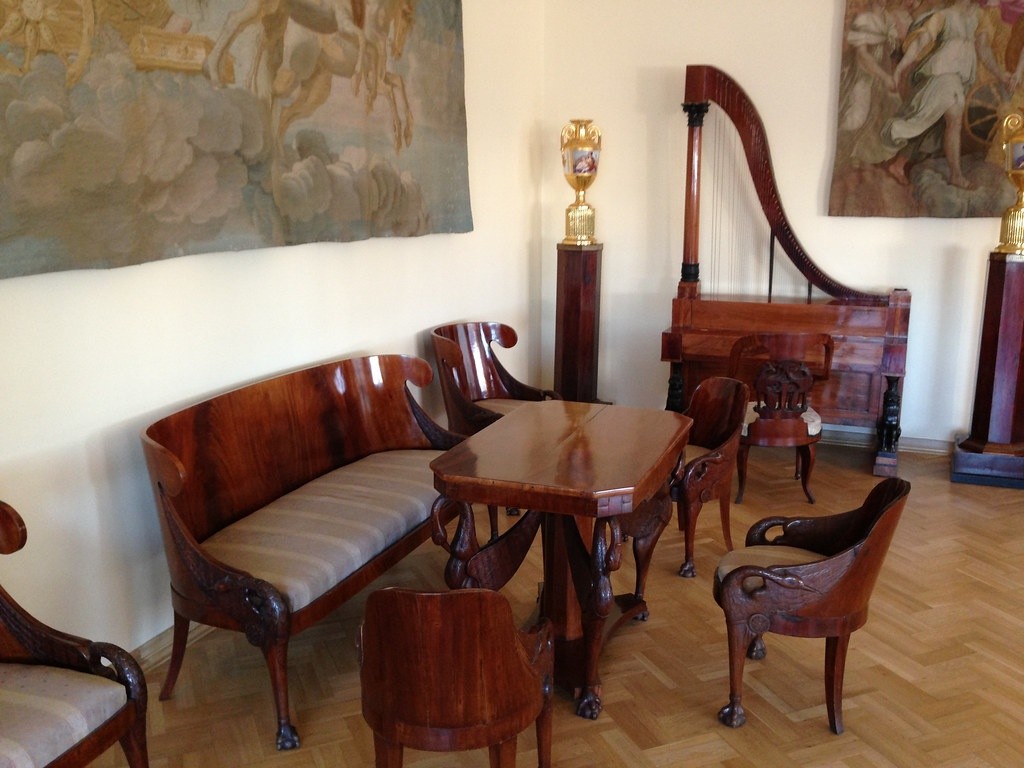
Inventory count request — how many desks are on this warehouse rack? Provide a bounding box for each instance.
[430,400,693,720]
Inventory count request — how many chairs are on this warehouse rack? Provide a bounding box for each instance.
[666,377,749,577]
[728,332,835,506]
[715,477,912,730]
[358,587,554,768]
[430,322,562,518]
[0,501,152,768]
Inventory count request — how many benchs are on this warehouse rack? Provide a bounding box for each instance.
[140,355,479,750]
